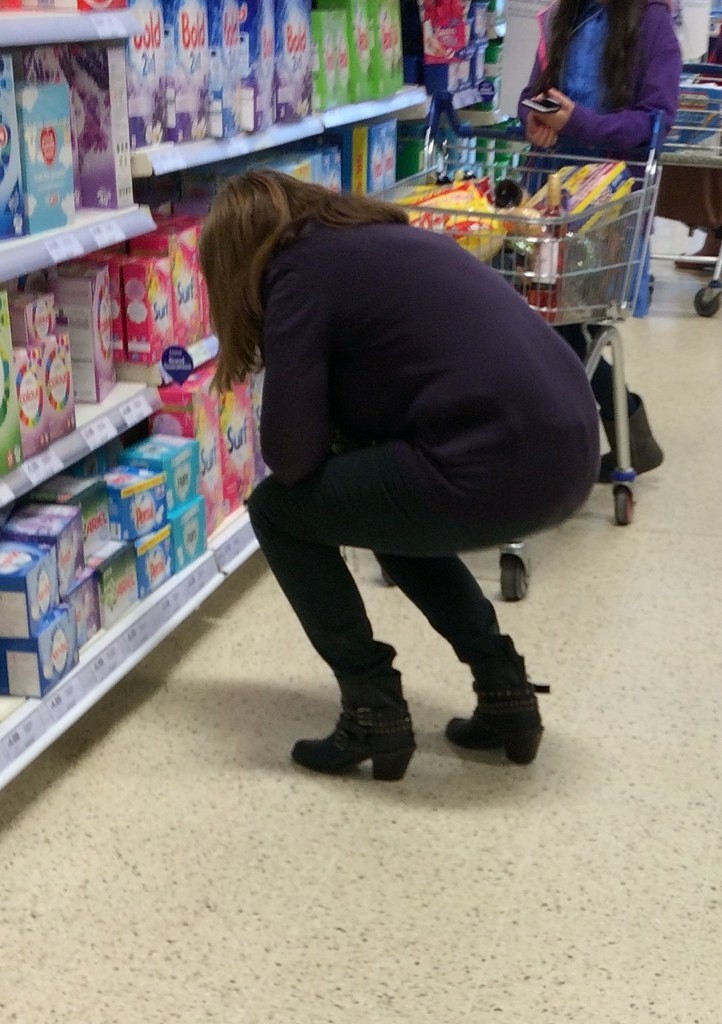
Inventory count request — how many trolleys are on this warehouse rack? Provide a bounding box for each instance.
[365,88,666,600]
[649,60,721,317]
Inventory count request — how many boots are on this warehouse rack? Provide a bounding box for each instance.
[598,393,665,481]
[675,230,722,271]
[291,671,418,782]
[444,657,549,762]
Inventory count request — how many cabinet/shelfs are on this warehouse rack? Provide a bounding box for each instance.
[0,0,428,799]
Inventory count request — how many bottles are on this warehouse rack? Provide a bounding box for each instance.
[528,175,569,322]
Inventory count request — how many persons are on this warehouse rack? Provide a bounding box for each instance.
[195,169,602,784]
[673,0,722,269]
[517,0,682,482]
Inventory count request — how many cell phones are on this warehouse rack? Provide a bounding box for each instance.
[521,96,562,113]
